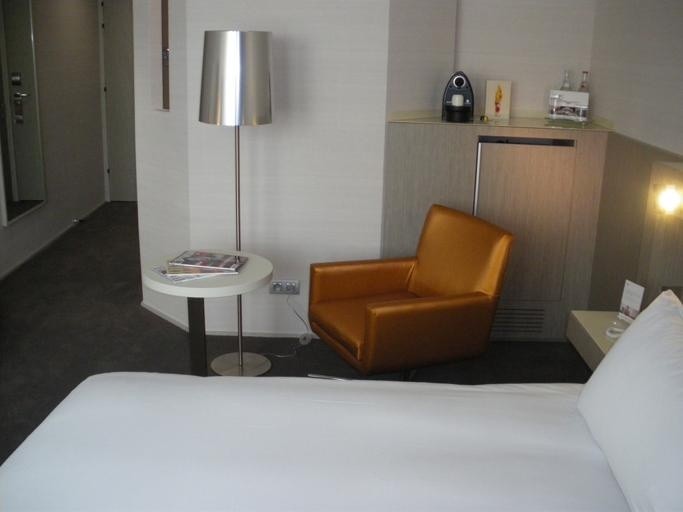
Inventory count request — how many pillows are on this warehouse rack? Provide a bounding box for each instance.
[575,289,683,507]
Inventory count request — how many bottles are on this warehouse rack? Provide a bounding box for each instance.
[578,71,590,91]
[560,67,573,90]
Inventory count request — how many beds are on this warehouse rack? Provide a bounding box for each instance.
[0,283,683,509]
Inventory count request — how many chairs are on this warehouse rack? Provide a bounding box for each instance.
[306,204,518,380]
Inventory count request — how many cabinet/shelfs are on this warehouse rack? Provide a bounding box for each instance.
[474,139,576,301]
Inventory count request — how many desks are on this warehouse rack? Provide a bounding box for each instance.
[139,245,273,375]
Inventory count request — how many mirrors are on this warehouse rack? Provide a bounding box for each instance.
[1,0,47,229]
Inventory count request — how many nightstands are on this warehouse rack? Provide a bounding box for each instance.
[563,309,633,376]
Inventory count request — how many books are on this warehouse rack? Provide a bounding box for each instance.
[152,250,249,283]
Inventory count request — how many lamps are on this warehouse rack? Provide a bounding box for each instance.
[195,27,272,376]
